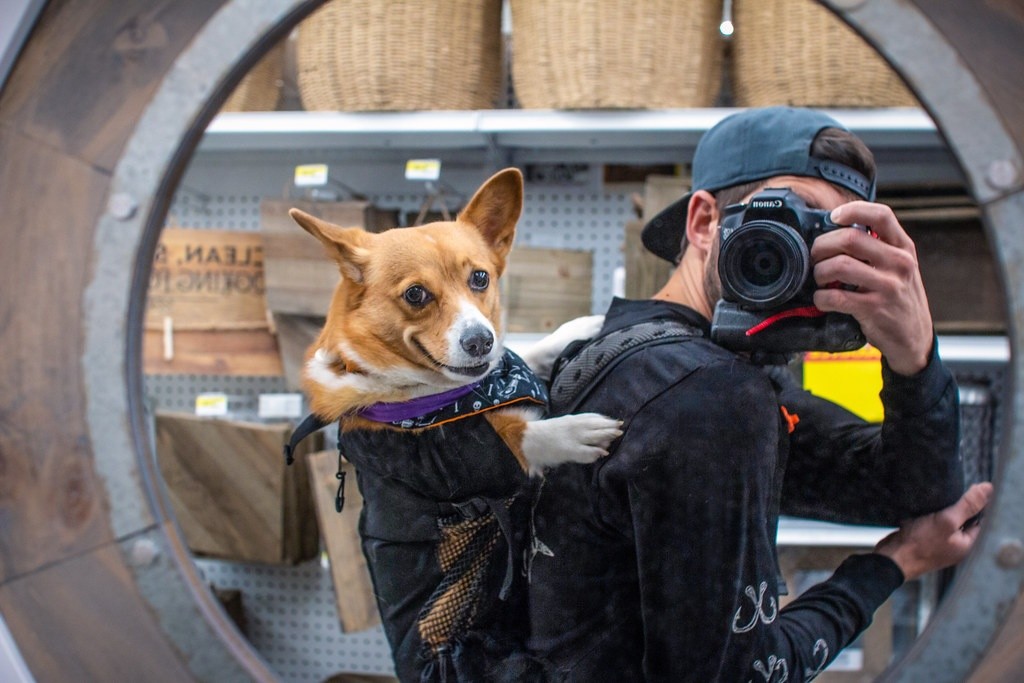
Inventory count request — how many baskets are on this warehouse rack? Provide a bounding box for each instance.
[511,0,727,110]
[219,69,281,111]
[294,0,509,112]
[733,1,917,108]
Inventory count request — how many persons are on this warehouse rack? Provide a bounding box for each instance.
[461,105,993,682]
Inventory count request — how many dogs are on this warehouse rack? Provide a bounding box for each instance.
[285,164,626,683]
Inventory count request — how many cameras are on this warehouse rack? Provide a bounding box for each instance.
[711,186,869,354]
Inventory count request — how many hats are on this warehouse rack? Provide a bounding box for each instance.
[640,104,878,270]
